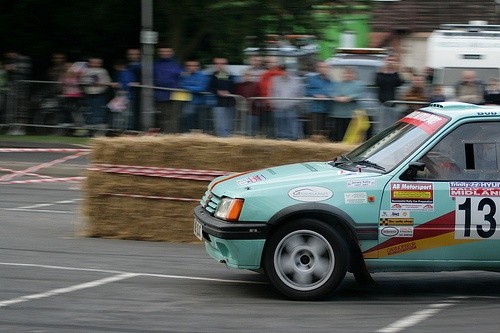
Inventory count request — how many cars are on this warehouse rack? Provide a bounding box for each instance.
[194,102,500,300]
[319,47,392,134]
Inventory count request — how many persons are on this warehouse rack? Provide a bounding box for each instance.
[0,46,496,142]
